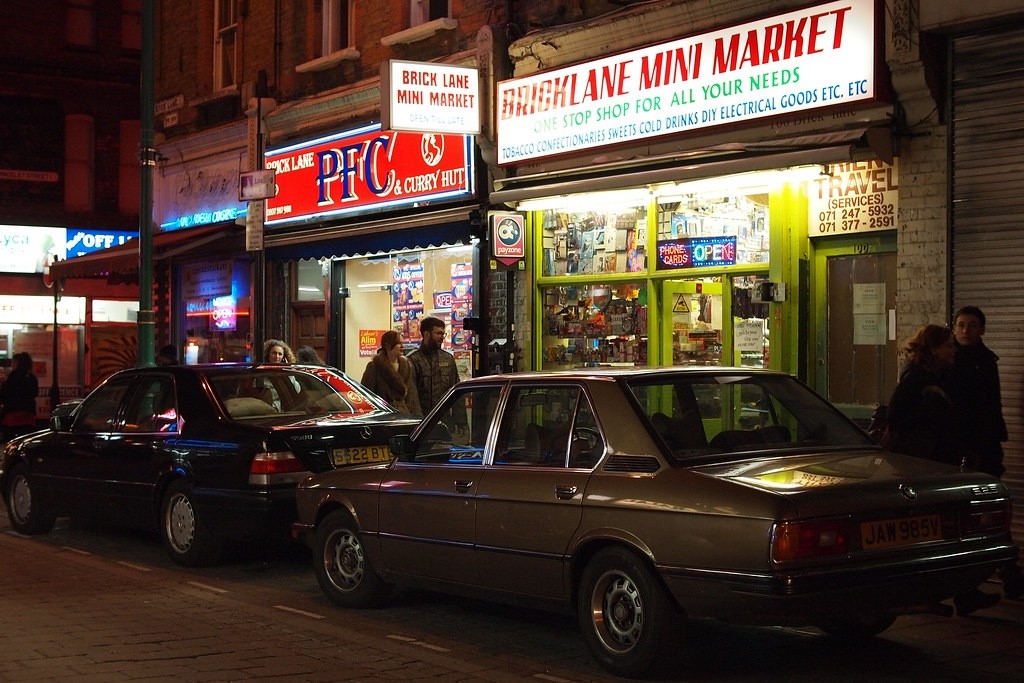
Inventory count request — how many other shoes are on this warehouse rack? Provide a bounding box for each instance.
[1000,567,1024,599]
[952,592,1000,618]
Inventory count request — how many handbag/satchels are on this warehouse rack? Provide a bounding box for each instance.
[867,404,890,444]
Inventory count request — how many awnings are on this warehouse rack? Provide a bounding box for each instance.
[50,225,226,281]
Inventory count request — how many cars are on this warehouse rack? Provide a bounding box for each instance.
[0,361,452,569]
[293,366,1021,679]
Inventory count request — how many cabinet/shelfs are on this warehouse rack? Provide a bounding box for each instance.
[559,287,723,418]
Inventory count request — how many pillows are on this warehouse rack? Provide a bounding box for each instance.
[315,391,371,411]
[223,398,277,416]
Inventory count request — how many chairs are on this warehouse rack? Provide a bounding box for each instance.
[652,409,708,450]
[138,391,174,424]
[525,422,577,462]
[242,388,273,407]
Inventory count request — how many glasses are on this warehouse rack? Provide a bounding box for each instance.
[428,330,447,339]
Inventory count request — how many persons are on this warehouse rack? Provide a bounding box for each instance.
[885,307,1024,618]
[152,339,332,413]
[696,315,709,330]
[0,352,39,443]
[362,331,423,417]
[405,316,469,442]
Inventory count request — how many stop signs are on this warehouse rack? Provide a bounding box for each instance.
[43,255,56,289]
